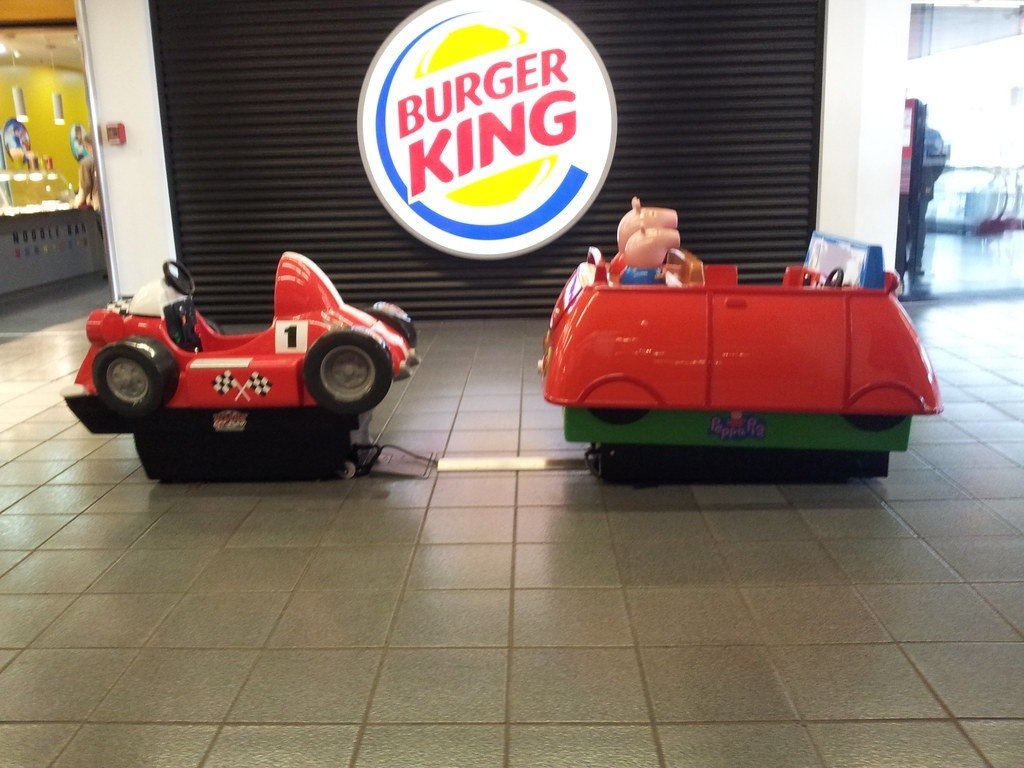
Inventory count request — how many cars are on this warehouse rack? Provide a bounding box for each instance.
[535,230,945,483]
[61,249,420,477]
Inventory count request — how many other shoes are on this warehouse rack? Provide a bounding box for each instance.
[910,279,931,294]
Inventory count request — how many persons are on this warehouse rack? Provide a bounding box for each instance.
[895,99,948,280]
[74,132,108,282]
